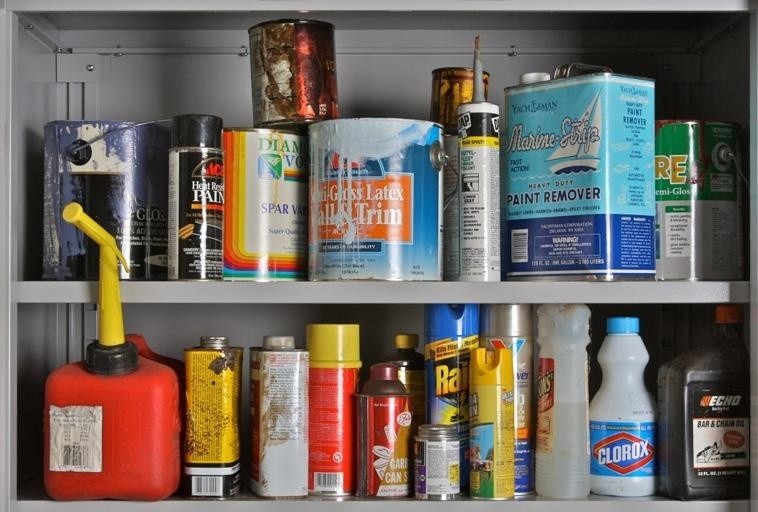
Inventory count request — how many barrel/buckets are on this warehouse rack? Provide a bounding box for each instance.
[41,335,183,501]
[658,305,752,499]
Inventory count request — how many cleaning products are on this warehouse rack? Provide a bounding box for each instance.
[469,346,516,500]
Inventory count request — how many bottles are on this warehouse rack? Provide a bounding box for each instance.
[356,363,408,498]
[383,333,425,421]
[445,39,503,281]
[166,112,224,280]
[246,334,311,499]
[304,323,365,499]
[409,423,462,501]
[182,333,245,499]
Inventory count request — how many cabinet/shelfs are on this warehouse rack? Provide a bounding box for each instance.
[0,0,758,510]
